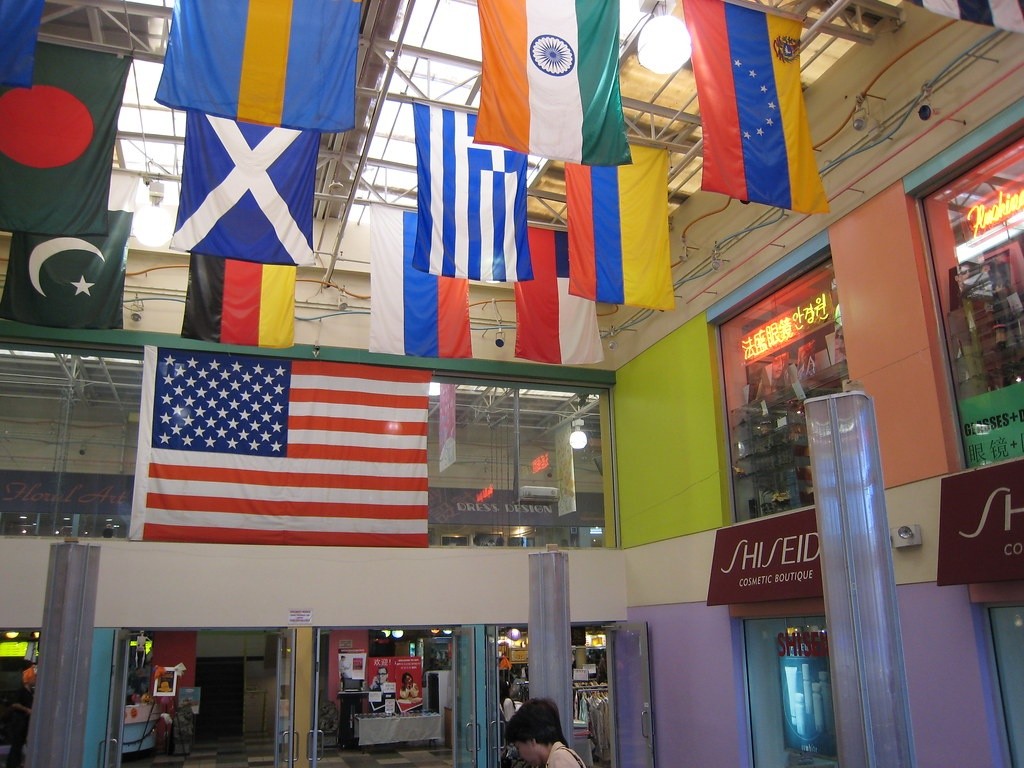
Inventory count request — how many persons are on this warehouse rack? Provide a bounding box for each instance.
[498,678,586,768]
[338,655,351,678]
[135,630,150,670]
[950,262,971,311]
[835,326,845,363]
[400,672,419,698]
[370,666,389,692]
[772,354,784,379]
[5,669,34,768]
[474,535,504,546]
[102,524,113,539]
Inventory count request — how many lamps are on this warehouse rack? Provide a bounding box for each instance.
[495,327,506,347]
[917,80,934,121]
[338,284,348,311]
[79,440,88,456]
[679,239,690,261]
[569,417,587,449]
[131,293,144,322]
[149,173,164,207]
[889,524,922,549]
[607,332,617,351]
[852,103,868,130]
[637,0,693,75]
[710,246,724,272]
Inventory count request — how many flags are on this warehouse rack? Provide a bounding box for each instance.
[905,0,1024,34]
[472,0,634,167]
[0,0,134,328]
[565,141,675,310]
[514,225,605,364]
[369,203,472,358]
[684,0,830,216]
[154,0,359,348]
[411,103,534,281]
[129,344,430,547]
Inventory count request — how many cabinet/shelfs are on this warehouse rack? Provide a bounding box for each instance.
[731,359,849,478]
[946,281,1024,386]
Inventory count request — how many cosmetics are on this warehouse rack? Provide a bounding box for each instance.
[785,664,832,735]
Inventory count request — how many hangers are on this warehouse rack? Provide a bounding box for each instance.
[574,681,608,700]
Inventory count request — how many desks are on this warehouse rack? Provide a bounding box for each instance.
[355,712,442,754]
[338,691,396,748]
